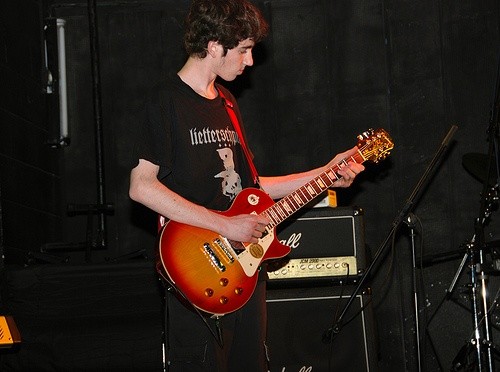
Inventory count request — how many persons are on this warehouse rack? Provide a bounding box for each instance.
[126,0,366,372]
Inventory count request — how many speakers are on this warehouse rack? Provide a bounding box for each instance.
[263,284,379,372]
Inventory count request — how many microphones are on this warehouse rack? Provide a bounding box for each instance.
[332,320,341,334]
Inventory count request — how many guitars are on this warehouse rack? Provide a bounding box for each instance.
[158,126,395,315]
